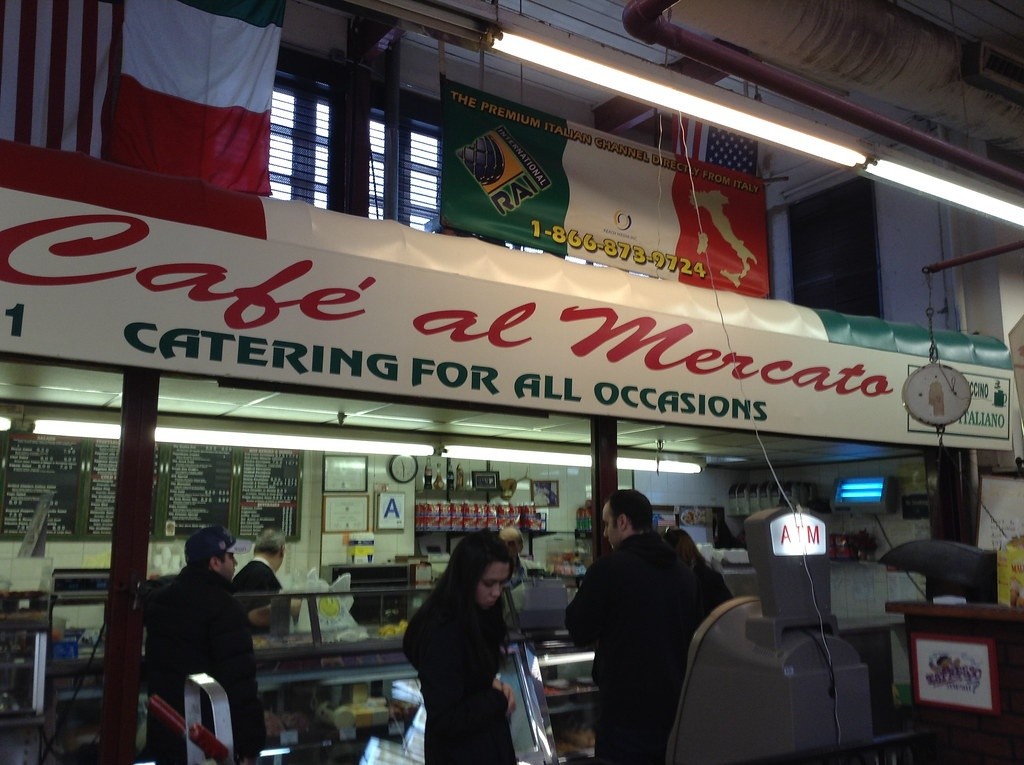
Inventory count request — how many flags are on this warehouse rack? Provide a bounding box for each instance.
[110,0,286,196]
[0,0,124,161]
[672,113,754,176]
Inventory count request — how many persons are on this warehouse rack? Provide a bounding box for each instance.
[499,527,527,578]
[231,526,302,634]
[663,529,734,623]
[402,529,516,765]
[564,490,704,765]
[143,526,267,765]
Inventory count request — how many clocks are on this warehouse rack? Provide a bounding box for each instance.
[389,455,417,484]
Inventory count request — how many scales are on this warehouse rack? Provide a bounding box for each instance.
[875,278,1009,606]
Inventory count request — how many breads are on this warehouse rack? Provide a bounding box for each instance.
[550,724,595,752]
[378,621,407,636]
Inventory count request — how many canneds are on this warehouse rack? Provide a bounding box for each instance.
[576,499,592,531]
[414,503,538,531]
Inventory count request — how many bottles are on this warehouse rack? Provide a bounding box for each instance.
[456,464,464,491]
[424,457,432,490]
[434,463,444,490]
[447,464,454,490]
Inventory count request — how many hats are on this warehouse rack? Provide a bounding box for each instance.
[185,526,252,563]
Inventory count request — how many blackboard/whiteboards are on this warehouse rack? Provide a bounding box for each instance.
[1,429,88,542]
[81,437,304,542]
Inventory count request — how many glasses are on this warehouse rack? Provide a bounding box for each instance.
[502,539,515,547]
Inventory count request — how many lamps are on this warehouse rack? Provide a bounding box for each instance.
[830,477,901,516]
[357,0,1024,233]
[0,401,705,476]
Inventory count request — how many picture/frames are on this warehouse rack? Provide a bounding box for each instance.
[472,472,500,491]
[530,480,560,508]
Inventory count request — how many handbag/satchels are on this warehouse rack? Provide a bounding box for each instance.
[504,571,568,631]
[296,568,358,631]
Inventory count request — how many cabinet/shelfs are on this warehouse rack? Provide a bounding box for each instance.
[258,632,594,765]
[415,489,558,539]
